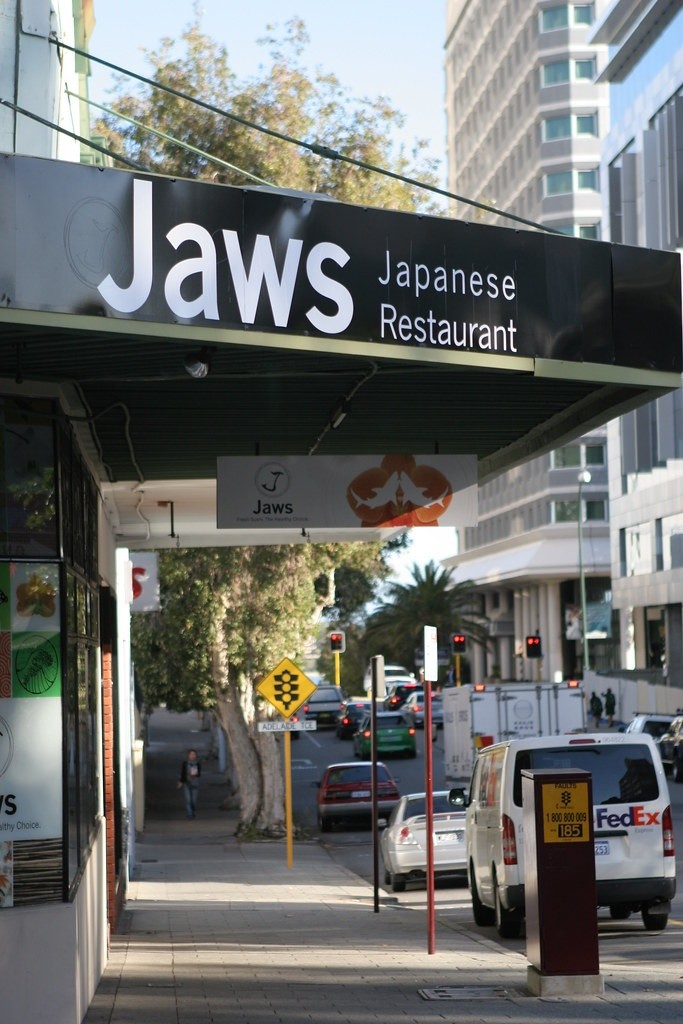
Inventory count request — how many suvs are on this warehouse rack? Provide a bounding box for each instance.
[619,712,678,775]
[385,682,425,718]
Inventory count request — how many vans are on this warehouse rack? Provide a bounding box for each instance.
[448,731,677,942]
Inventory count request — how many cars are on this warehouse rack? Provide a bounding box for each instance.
[311,761,400,832]
[301,685,345,731]
[336,701,389,740]
[363,665,419,698]
[654,714,682,782]
[397,691,445,729]
[375,790,471,893]
[352,712,422,763]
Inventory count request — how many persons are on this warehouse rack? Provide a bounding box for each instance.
[601,689,615,727]
[178,750,201,819]
[590,690,603,728]
[659,708,683,783]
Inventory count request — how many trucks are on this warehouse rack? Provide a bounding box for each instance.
[433,682,588,795]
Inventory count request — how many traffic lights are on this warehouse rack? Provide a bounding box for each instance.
[451,633,467,656]
[526,636,542,658]
[329,631,346,653]
